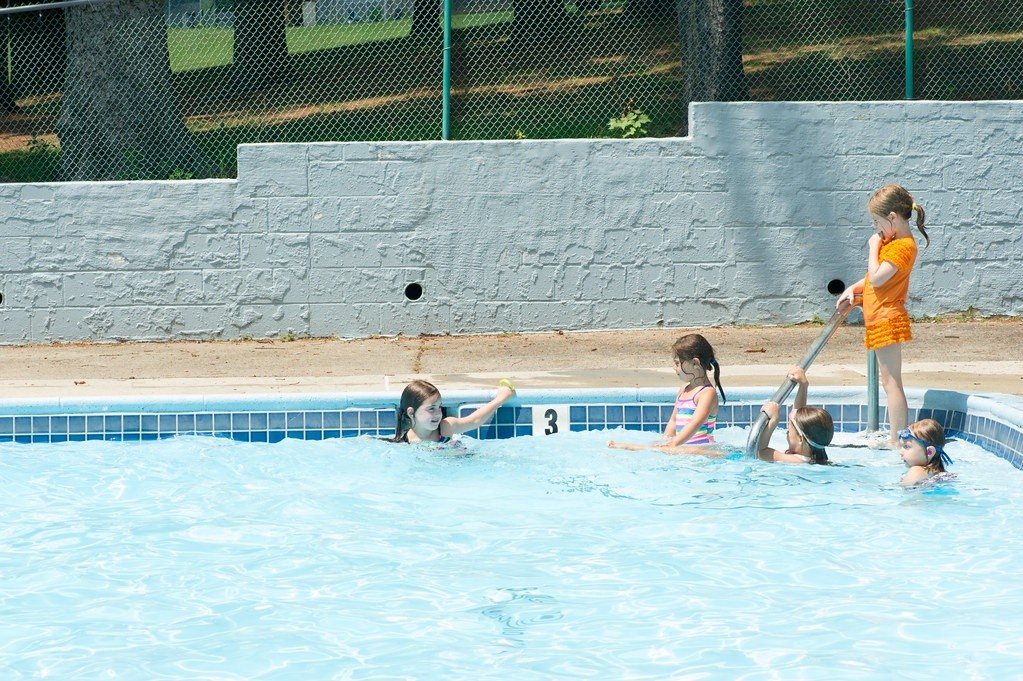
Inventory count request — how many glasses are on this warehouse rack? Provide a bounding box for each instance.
[788,408,798,420]
[896,427,913,440]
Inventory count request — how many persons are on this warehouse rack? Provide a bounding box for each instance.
[395,380,515,443]
[664,334,726,445]
[835,184,929,440]
[607,366,834,464]
[896,419,953,482]
